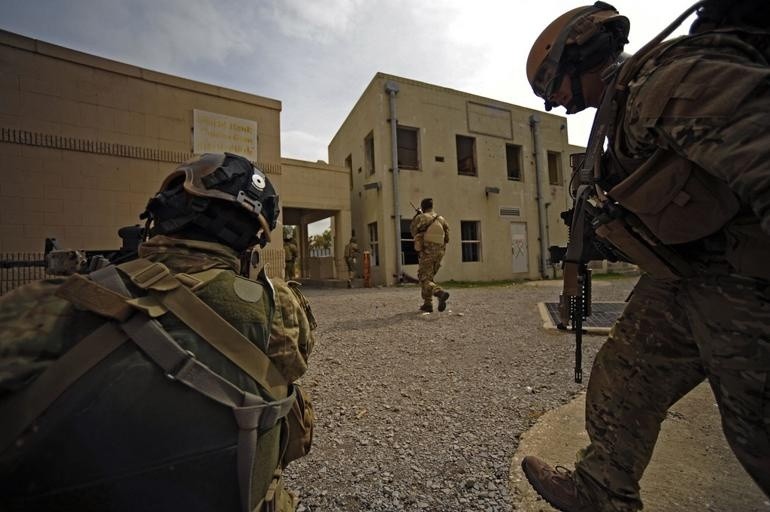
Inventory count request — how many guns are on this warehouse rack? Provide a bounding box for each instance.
[547,154,595,383]
[409,201,422,215]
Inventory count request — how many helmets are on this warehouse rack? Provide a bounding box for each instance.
[525,0,630,98]
[139,151,280,243]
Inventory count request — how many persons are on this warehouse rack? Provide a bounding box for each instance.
[522,0,770,512]
[344,237,361,289]
[283,233,298,282]
[409,199,450,312]
[0,151,317,512]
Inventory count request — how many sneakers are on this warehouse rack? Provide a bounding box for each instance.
[520,455,595,512]
[437,291,450,312]
[419,303,433,312]
